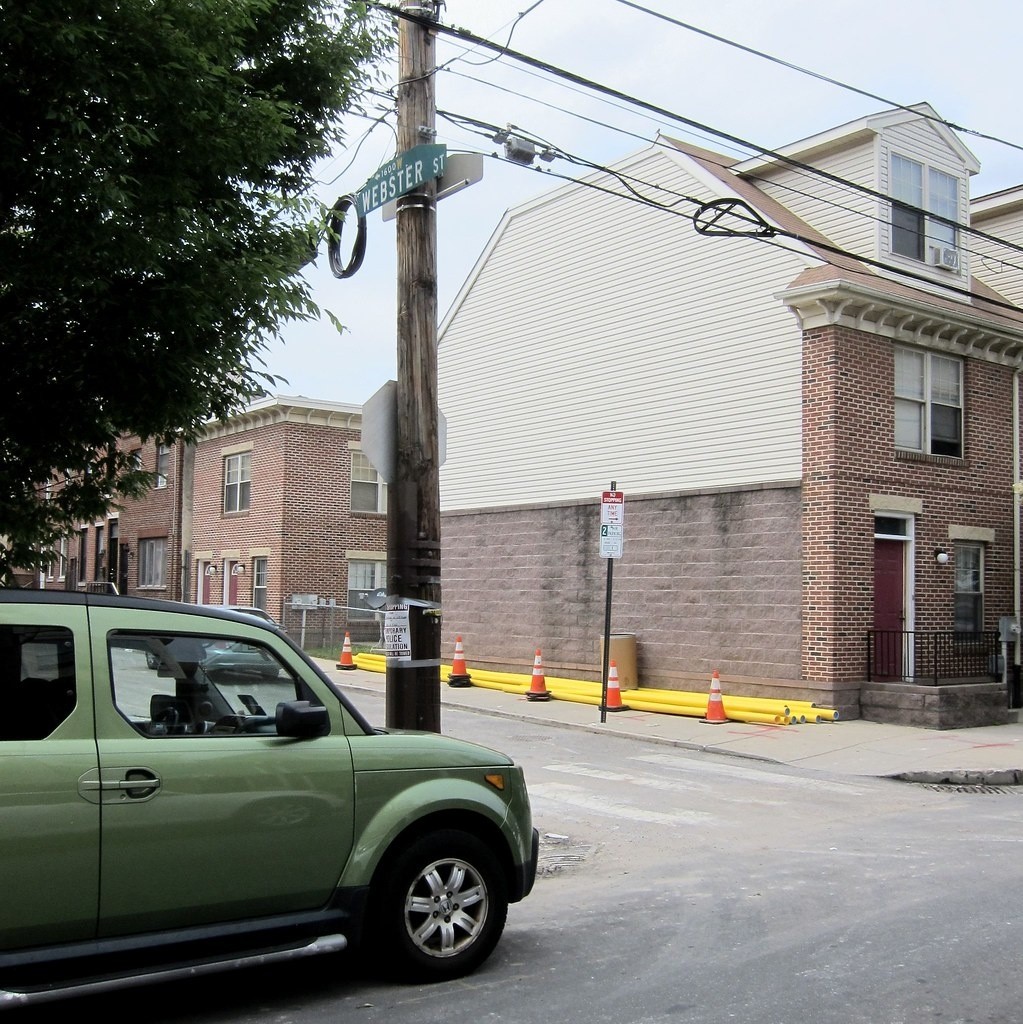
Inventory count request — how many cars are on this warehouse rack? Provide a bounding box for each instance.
[143,603,287,683]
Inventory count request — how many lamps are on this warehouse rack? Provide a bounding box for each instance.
[99,551,106,559]
[124,548,134,560]
[237,565,245,572]
[934,548,948,563]
[209,566,218,573]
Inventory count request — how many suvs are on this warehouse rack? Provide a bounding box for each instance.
[0,583,544,1024]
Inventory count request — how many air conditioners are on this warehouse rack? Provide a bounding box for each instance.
[932,246,959,271]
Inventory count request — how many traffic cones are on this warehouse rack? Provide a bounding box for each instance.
[699,668,732,724]
[335,631,357,670]
[598,659,631,712]
[525,648,552,702]
[447,635,471,688]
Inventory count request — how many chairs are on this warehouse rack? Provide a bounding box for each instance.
[14,676,62,739]
